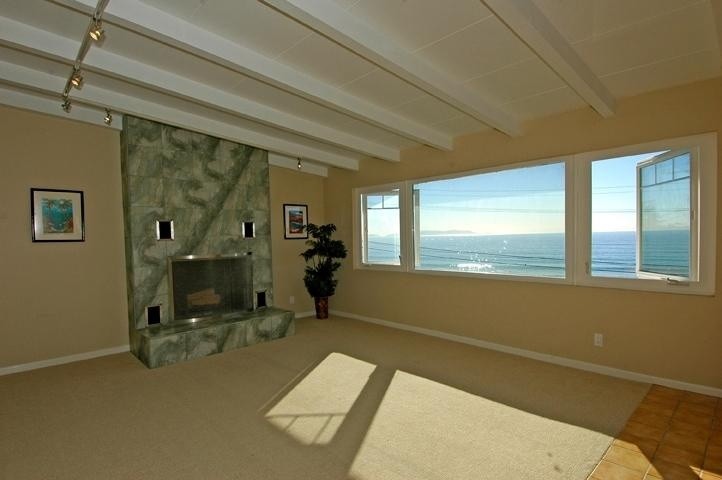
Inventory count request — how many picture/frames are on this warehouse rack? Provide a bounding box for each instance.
[283,204,308,239]
[30,187,85,242]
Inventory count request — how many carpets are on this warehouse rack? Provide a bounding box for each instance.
[0,313,654,480]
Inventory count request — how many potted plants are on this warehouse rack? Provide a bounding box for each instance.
[299,223,348,319]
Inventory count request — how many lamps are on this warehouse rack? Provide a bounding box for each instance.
[72,76,83,86]
[104,114,113,125]
[90,27,105,40]
